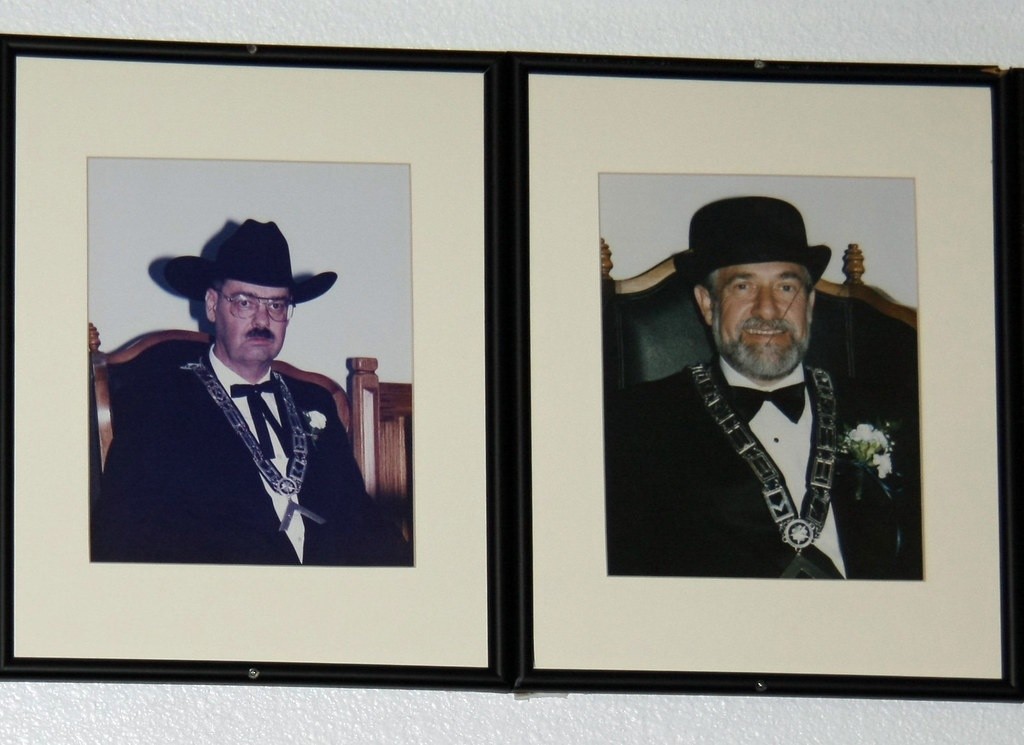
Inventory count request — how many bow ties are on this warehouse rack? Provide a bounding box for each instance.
[728,381,807,425]
[230,379,297,459]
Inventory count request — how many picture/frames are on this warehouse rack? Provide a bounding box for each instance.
[0,33,513,696]
[512,49,1024,705]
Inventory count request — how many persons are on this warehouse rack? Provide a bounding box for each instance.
[605,196,923,581]
[90,218,405,568]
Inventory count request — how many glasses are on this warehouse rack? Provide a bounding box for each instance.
[215,290,295,322]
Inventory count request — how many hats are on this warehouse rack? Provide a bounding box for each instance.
[672,195,832,284]
[164,219,339,305]
